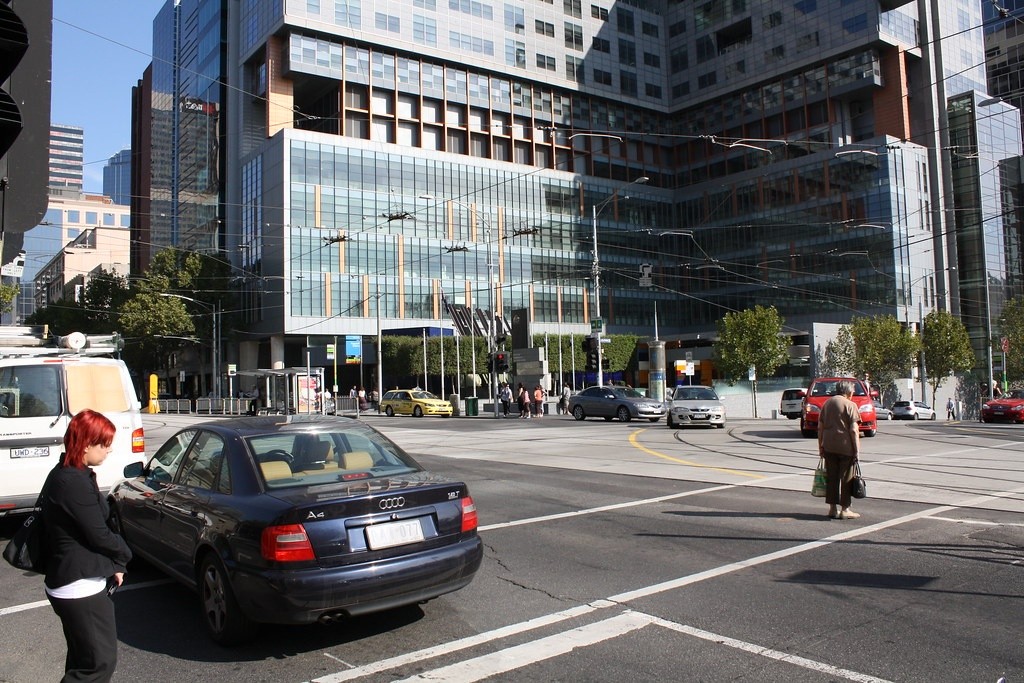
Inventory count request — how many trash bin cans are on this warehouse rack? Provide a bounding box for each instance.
[466,397,478,416]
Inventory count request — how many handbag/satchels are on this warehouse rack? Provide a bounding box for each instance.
[851,462,866,499]
[2,510,48,575]
[811,459,827,497]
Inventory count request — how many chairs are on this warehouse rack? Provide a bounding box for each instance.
[199,455,229,485]
[338,451,374,469]
[294,440,338,472]
[260,460,292,481]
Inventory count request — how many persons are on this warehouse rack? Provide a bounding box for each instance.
[207,389,212,398]
[249,386,259,415]
[607,379,613,386]
[981,380,1002,399]
[38,409,133,683]
[666,383,673,401]
[946,398,955,421]
[497,382,548,419]
[350,384,390,411]
[818,379,861,520]
[562,382,571,415]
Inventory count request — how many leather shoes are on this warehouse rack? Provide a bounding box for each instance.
[829,509,841,519]
[840,509,860,519]
